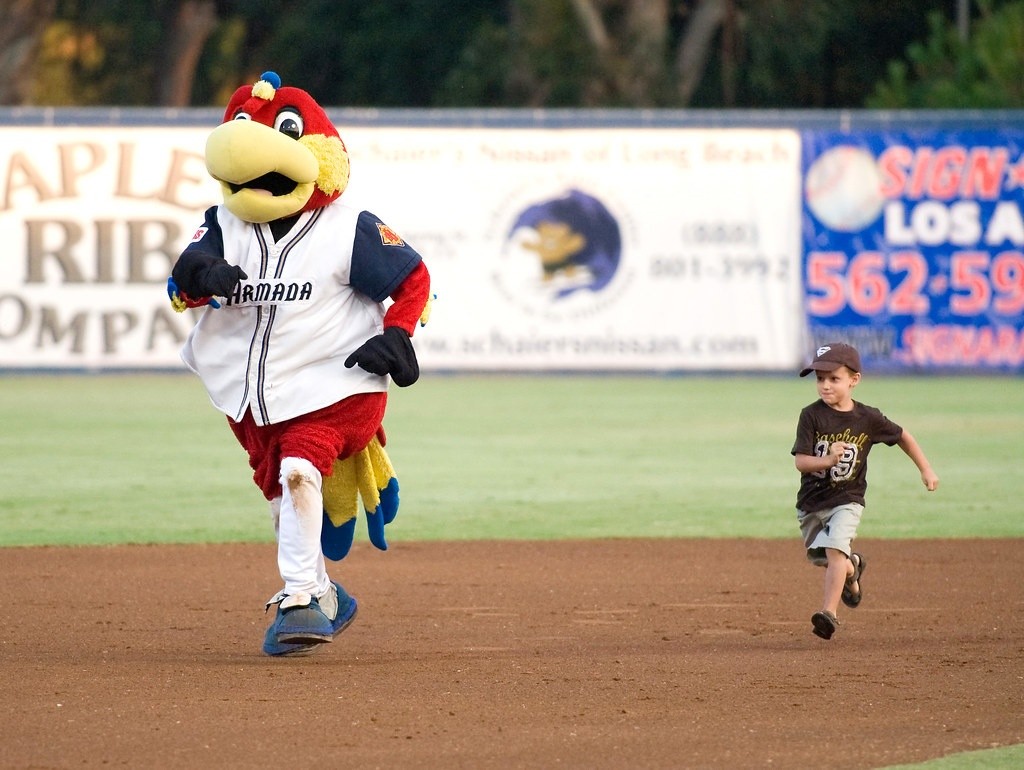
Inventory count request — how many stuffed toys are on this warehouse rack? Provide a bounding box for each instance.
[172,73,429,657]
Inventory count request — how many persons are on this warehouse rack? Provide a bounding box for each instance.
[791,344,940,640]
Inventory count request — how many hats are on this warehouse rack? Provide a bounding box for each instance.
[800,343,860,377]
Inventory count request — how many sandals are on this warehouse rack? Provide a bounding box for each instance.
[811,609,840,640]
[841,553,866,608]
